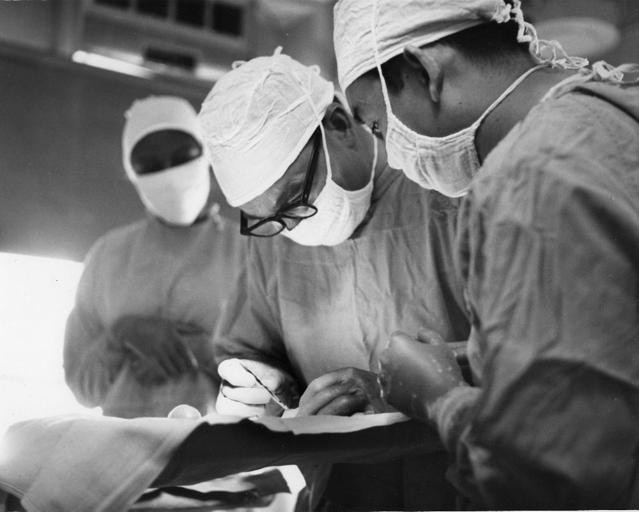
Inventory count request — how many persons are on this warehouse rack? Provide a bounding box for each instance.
[332,0,638,510]
[196,44,465,511]
[63,94,248,418]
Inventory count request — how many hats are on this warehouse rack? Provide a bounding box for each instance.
[191,53,337,209]
[333,1,522,105]
[120,96,206,176]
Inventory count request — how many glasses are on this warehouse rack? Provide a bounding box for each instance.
[239,126,324,238]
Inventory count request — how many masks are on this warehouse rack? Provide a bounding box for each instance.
[272,116,377,247]
[131,155,211,227]
[371,62,550,198]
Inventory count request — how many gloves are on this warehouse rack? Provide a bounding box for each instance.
[215,354,299,422]
[296,365,401,423]
[376,327,485,448]
